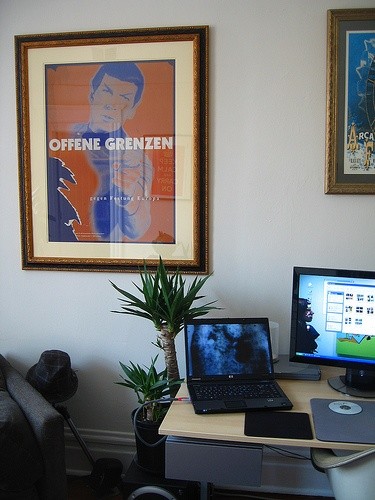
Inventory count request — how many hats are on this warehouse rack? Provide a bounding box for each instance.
[24,350,78,402]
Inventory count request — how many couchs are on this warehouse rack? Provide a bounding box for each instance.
[0,353,68,500]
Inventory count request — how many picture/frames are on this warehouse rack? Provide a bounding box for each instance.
[325,8,375,195]
[14,25,210,275]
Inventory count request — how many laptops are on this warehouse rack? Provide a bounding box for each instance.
[183,317,293,415]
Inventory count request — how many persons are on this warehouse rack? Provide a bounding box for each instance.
[295,297,319,354]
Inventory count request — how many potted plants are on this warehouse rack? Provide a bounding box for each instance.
[115,257,222,473]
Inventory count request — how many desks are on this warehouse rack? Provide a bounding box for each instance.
[157,356,375,500]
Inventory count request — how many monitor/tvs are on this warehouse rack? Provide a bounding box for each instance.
[290,267,375,398]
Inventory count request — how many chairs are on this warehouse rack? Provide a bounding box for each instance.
[310,447,375,500]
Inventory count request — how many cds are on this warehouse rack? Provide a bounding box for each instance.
[328,401,362,414]
